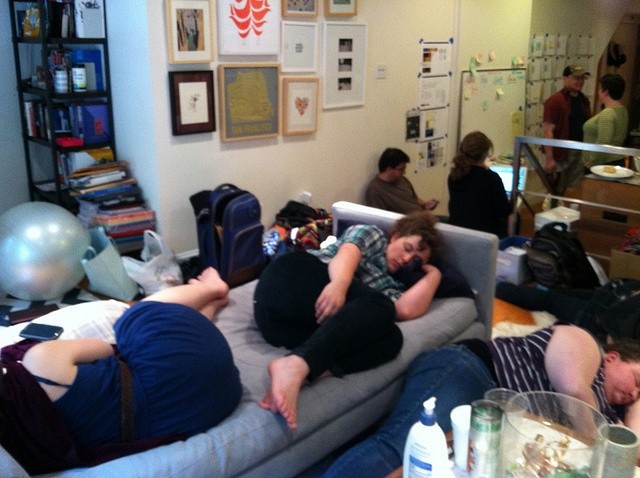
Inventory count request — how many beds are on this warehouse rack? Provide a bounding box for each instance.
[0,201,498,477]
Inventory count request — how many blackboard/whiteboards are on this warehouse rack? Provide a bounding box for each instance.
[457,68,526,160]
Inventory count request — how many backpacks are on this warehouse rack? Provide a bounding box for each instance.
[525,220,601,299]
[196,183,267,289]
[594,274,640,338]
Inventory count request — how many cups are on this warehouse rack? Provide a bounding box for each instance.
[484,388,530,452]
[497,385,612,477]
[588,424,640,478]
[450,405,472,471]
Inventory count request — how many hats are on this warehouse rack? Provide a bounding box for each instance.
[563,64,592,78]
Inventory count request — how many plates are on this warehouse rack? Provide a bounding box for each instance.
[590,165,634,179]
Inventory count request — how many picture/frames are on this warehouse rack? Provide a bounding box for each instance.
[169,70,216,135]
[280,22,319,72]
[322,0,358,16]
[216,1,282,58]
[282,0,320,18]
[279,75,321,137]
[321,21,367,110]
[216,63,281,142]
[164,0,217,64]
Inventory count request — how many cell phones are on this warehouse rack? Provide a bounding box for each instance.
[20,323,61,341]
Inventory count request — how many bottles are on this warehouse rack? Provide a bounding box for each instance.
[465,399,505,478]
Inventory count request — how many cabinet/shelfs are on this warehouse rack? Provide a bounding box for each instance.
[8,0,119,206]
[582,175,640,268]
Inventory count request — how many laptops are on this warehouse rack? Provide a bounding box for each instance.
[489,163,527,212]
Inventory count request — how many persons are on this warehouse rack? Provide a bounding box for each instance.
[1,266,243,477]
[446,132,520,241]
[366,147,440,216]
[253,210,442,432]
[581,74,630,184]
[542,65,592,195]
[322,322,640,477]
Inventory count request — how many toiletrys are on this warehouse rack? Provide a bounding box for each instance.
[403,397,449,478]
